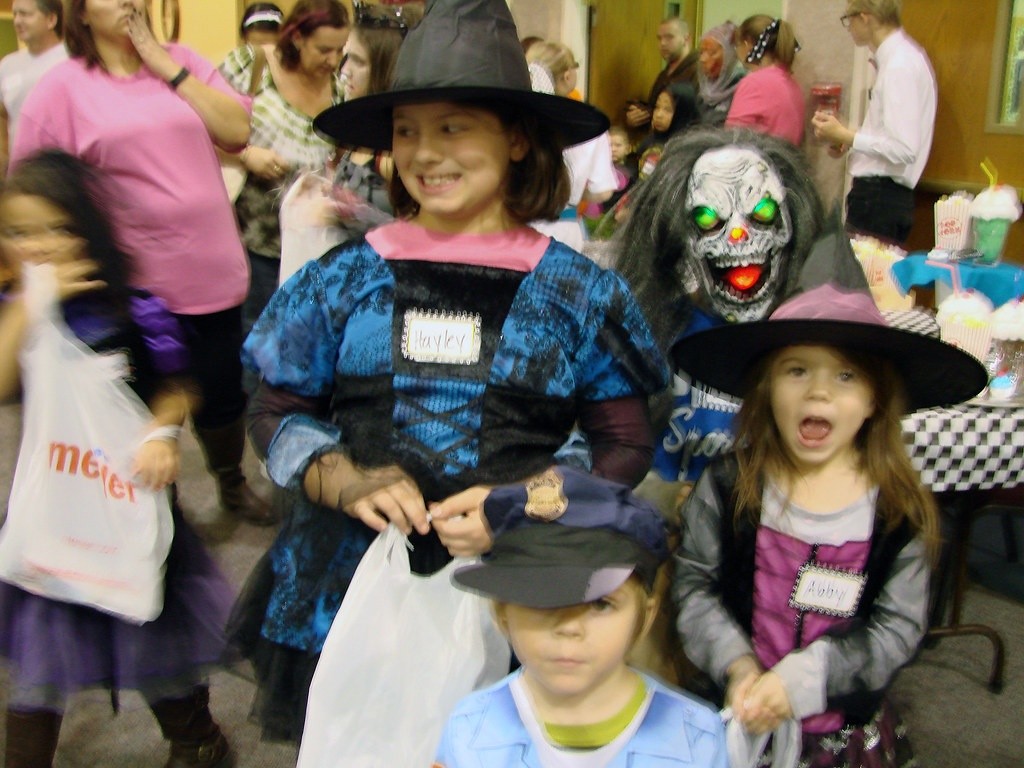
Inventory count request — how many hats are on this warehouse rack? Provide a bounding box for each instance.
[312,0,610,161]
[450,465,668,609]
[668,286,989,412]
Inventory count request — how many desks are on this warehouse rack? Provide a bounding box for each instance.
[669,305,1024,694]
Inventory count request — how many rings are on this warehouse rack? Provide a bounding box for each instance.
[274,164,280,173]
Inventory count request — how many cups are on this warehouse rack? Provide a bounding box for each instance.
[973,216,1012,264]
[934,189,974,260]
[812,84,842,118]
[940,315,991,363]
[983,337,1024,403]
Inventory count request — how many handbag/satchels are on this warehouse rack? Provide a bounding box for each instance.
[213,46,265,207]
[0,265,175,623]
[296,508,510,768]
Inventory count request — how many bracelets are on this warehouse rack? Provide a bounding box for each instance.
[138,423,180,444]
[169,67,191,90]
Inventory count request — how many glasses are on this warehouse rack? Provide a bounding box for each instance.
[840,12,862,27]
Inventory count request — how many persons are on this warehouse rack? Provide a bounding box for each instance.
[633,81,695,174]
[600,126,636,213]
[693,20,748,130]
[647,130,849,482]
[670,282,989,768]
[232,0,431,482]
[436,465,729,768]
[0,0,76,260]
[722,15,806,151]
[241,0,672,768]
[518,35,617,255]
[812,0,938,249]
[0,147,225,768]
[623,17,702,140]
[16,0,281,574]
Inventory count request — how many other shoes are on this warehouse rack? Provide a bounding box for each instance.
[213,478,277,524]
[163,727,230,768]
